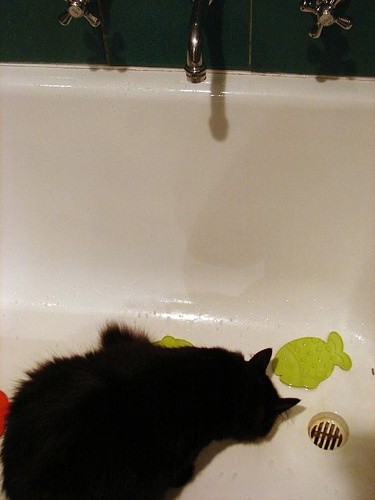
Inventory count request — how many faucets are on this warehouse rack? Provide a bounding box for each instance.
[184,1,223,85]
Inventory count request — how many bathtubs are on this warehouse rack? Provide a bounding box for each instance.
[1,62,375,499]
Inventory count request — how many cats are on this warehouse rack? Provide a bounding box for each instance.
[1,319,301,499]
